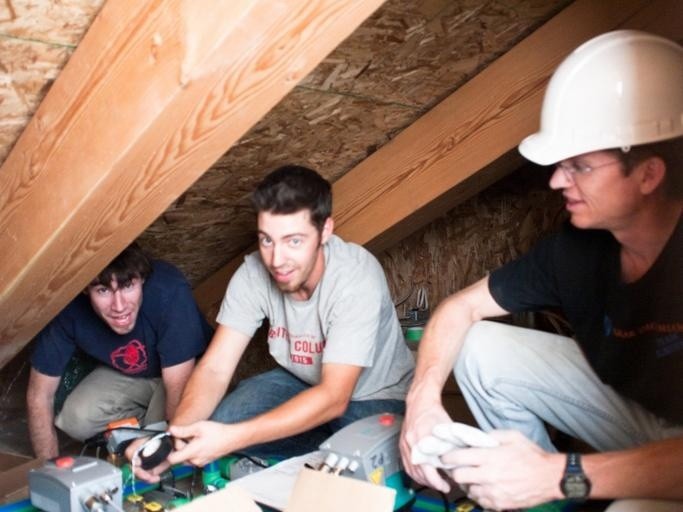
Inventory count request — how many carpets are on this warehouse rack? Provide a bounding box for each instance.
[0,419,615,512]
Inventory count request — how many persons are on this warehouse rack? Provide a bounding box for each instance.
[125,164,416,483]
[25,240,215,463]
[397,30,683,512]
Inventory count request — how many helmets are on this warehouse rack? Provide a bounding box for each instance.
[519,30,681,165]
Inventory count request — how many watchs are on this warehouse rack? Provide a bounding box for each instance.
[560,451,592,502]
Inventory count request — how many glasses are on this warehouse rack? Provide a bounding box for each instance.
[563,158,594,175]
